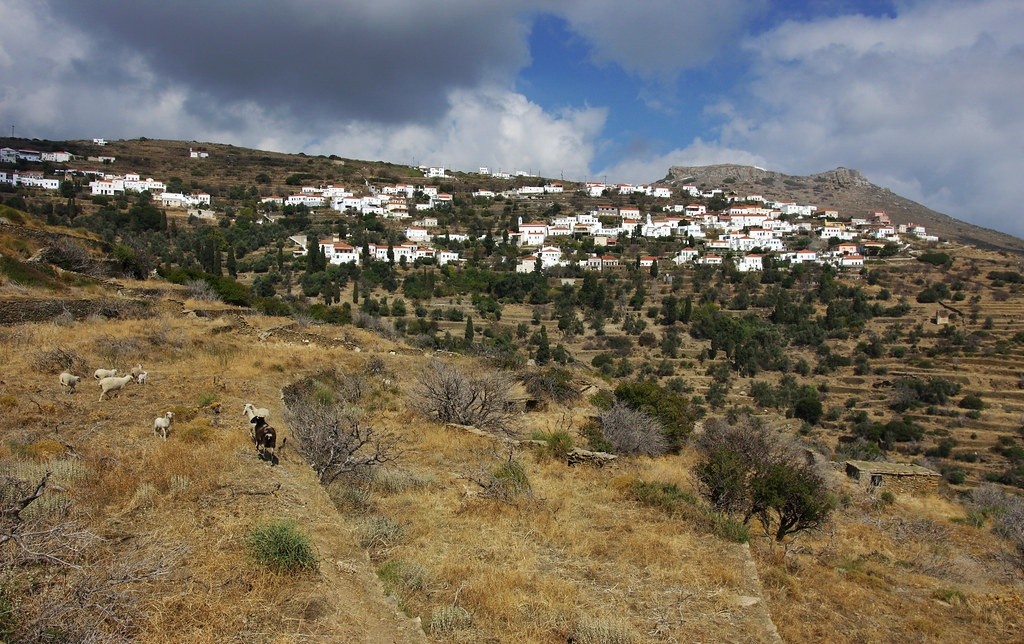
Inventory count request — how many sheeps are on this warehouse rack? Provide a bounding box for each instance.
[153,412,175,442]
[94,363,148,402]
[242,404,277,466]
[59,373,81,395]
